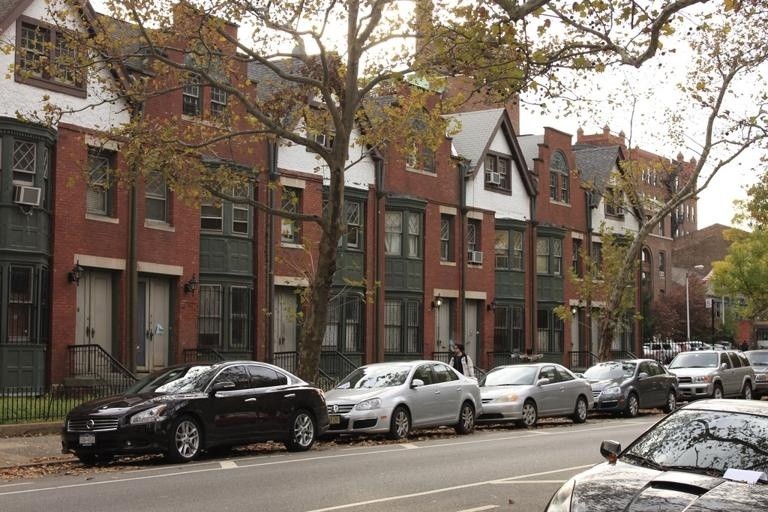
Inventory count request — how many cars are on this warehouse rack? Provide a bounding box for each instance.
[544,397,768,512]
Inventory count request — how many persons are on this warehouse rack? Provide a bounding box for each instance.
[449,344,474,379]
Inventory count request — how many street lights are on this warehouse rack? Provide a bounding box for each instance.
[685,263,705,341]
[436,296,444,353]
